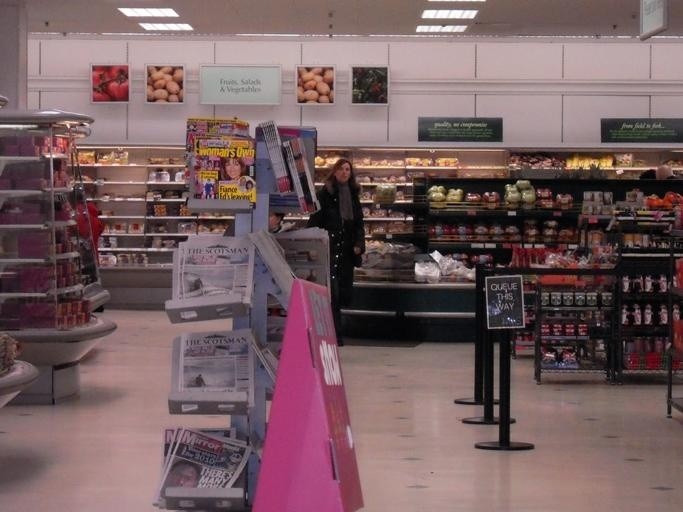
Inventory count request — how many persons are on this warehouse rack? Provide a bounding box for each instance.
[303,159,366,347]
[73,184,104,313]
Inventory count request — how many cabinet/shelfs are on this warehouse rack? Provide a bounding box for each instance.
[0,115,119,404]
[137,193,332,512]
[71,139,237,289]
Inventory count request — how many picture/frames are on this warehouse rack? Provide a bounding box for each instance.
[196,63,283,106]
[293,62,336,108]
[142,63,187,106]
[346,63,391,107]
[89,62,132,106]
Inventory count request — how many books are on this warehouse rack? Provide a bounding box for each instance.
[149,117,332,512]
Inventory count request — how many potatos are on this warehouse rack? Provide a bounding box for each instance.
[147,66,183,103]
[298,67,334,103]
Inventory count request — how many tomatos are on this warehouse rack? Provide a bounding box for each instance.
[92,65,129,102]
